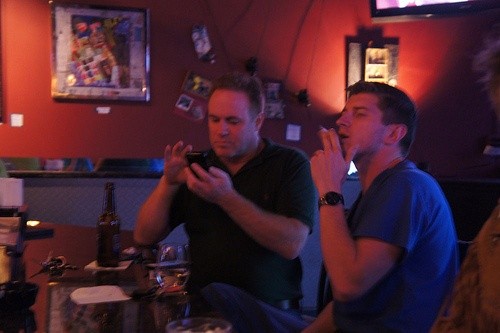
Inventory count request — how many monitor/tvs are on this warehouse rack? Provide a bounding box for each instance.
[370,0,500,17]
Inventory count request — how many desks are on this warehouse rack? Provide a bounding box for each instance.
[0,219,195,332]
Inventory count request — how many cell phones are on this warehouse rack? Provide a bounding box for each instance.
[185,151,209,178]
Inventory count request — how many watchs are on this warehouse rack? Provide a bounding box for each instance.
[315,190,346,210]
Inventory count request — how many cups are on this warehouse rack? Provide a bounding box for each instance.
[164,317,232,333]
[157,242,188,292]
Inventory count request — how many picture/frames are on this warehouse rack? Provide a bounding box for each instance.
[51,1,150,99]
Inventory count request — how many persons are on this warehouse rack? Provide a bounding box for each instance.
[132,73,316,317]
[185,78,459,333]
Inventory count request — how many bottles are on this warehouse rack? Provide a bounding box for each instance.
[96,183,122,267]
